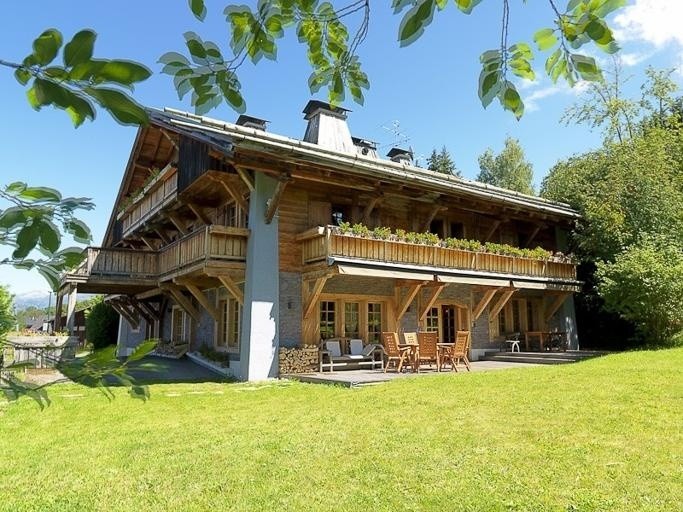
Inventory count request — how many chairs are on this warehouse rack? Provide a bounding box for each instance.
[504,333,524,352]
[383,330,468,371]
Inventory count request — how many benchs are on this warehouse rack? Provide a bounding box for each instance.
[314,336,385,372]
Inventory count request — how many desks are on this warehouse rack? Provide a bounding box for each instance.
[525,331,567,352]
